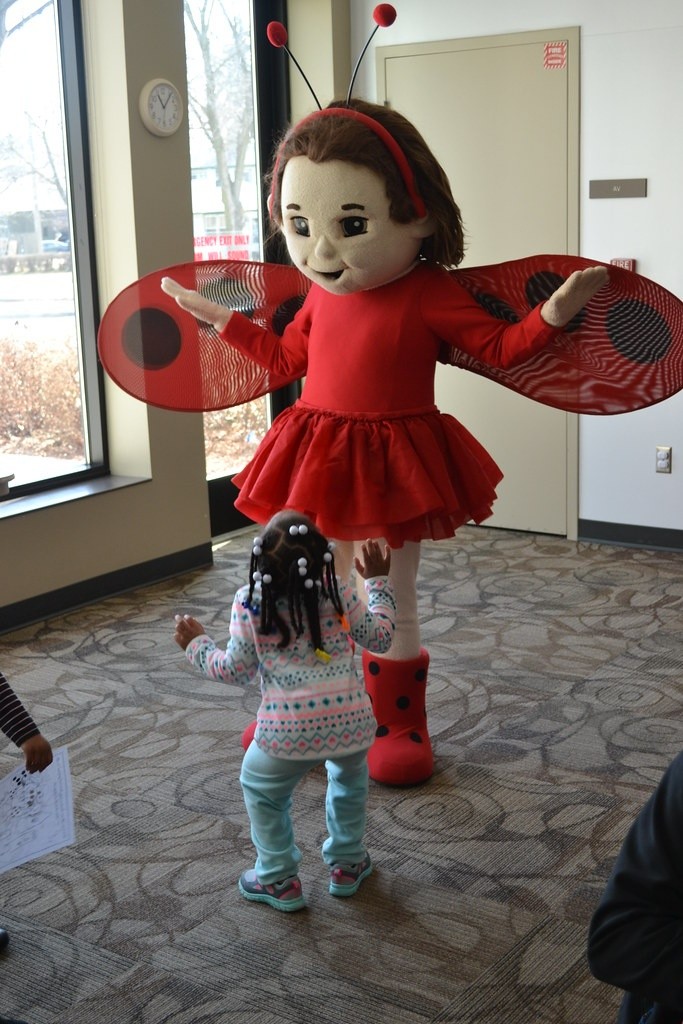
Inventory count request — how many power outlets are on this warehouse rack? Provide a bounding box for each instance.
[656,446,671,474]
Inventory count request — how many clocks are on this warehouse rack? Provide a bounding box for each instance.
[138,77,185,138]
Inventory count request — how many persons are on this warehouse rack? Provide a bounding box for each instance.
[0,672,53,957]
[173,510,396,911]
[587,749,683,1024]
[158,97,611,790]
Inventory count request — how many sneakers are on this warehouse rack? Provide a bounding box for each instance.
[328,848,373,897]
[238,868,306,912]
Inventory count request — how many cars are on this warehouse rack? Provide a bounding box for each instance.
[42,240,69,251]
